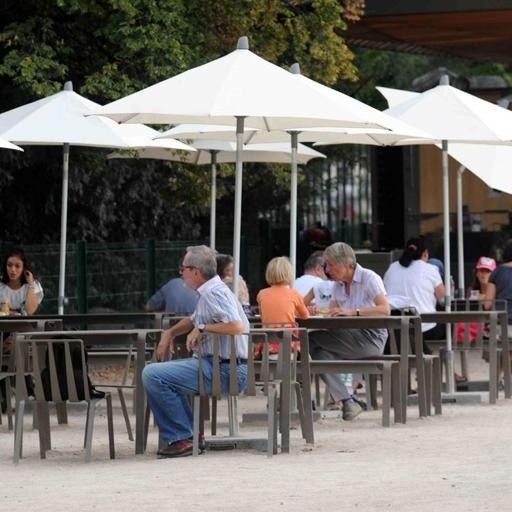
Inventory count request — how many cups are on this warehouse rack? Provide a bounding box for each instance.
[471,289,480,300]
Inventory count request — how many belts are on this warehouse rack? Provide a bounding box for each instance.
[207,354,248,366]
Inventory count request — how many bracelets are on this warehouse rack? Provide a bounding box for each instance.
[28,285,34,289]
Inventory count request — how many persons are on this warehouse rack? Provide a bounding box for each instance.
[215,253,250,316]
[140,244,251,458]
[0,247,44,414]
[144,274,202,361]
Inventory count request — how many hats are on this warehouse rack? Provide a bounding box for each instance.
[476,256,497,272]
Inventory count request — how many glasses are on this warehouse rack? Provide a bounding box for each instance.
[178,265,195,274]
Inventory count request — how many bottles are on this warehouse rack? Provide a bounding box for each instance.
[0,302,10,315]
[450,276,454,300]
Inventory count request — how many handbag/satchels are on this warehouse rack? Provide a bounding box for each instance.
[454,321,489,346]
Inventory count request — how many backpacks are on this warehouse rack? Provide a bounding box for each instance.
[40,332,106,401]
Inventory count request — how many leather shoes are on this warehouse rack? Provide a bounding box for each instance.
[343,400,363,421]
[454,374,469,387]
[157,437,201,458]
[196,434,206,450]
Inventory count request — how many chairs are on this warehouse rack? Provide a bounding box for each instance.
[192,332,278,457]
[13,338,115,461]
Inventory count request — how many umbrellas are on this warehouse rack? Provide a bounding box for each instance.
[106,125,327,252]
[1,133,25,153]
[0,79,196,316]
[149,62,436,285]
[83,34,394,302]
[373,81,512,298]
[311,74,512,350]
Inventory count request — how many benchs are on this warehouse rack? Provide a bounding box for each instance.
[241,300,512,427]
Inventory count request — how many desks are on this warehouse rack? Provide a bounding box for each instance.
[1,308,314,452]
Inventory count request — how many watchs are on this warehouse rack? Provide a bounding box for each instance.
[198,324,205,333]
